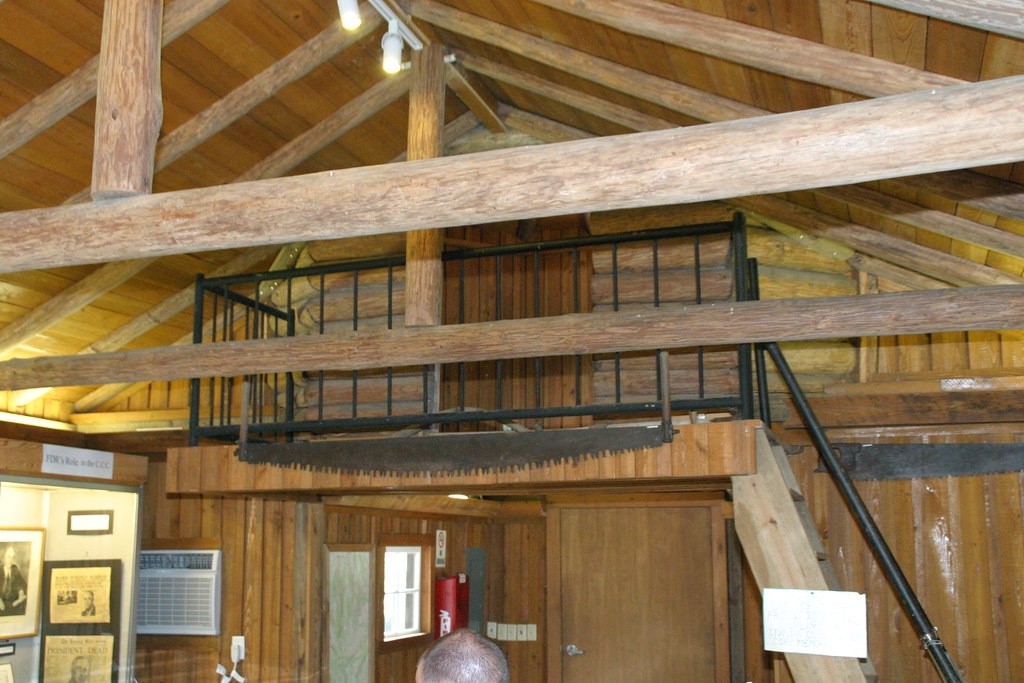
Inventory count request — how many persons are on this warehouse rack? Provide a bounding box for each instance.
[415,628,510,683]
[0,564,97,683]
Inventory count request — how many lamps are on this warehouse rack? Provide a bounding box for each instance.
[337,0,361,29]
[382,19,405,74]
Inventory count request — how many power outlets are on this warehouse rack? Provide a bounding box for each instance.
[232,636,245,660]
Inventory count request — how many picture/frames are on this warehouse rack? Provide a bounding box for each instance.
[0,527,47,638]
